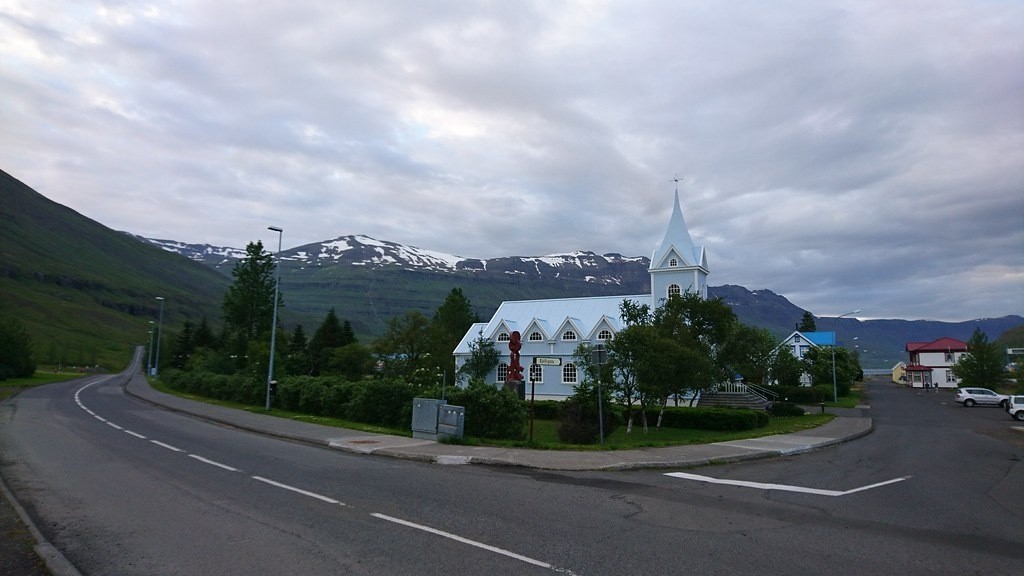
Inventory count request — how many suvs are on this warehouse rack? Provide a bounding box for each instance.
[1007,395,1024,422]
[955,387,1010,408]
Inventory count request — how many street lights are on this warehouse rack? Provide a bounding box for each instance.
[153,296,165,387]
[265,225,282,412]
[147,320,155,376]
[832,308,862,405]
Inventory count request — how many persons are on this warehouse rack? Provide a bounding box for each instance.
[925,383,930,390]
[934,382,938,389]
